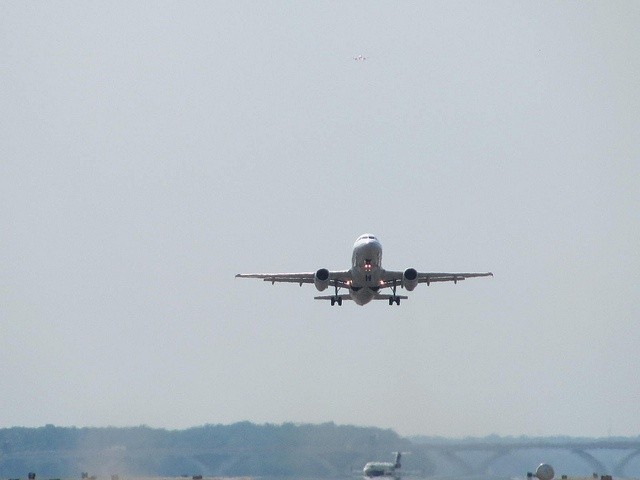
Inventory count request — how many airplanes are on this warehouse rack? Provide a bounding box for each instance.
[350,451,427,480]
[234,233,495,307]
